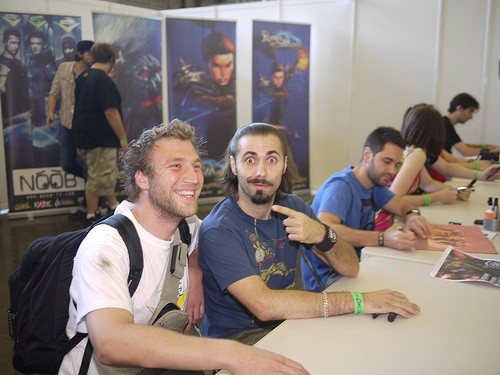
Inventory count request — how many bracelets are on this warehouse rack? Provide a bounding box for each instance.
[424,193,430,205]
[379,232,384,246]
[120,137,128,142]
[473,160,479,169]
[473,170,478,179]
[482,143,487,149]
[351,290,364,314]
[323,290,329,317]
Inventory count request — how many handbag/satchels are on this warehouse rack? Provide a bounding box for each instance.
[151,302,190,334]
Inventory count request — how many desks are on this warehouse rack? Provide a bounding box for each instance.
[213,162,500,375]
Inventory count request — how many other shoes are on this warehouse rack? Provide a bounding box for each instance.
[107,208,115,214]
[85,214,102,225]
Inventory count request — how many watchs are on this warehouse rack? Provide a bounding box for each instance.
[317,222,337,253]
[406,209,421,215]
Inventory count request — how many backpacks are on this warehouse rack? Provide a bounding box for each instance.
[8,219,190,375]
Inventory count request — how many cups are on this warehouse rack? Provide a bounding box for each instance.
[456,187,474,201]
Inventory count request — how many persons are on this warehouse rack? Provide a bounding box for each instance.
[402,103,500,182]
[57,118,311,375]
[0,29,75,167]
[301,127,431,292]
[375,107,472,231]
[46,41,111,217]
[443,93,500,156]
[198,123,420,373]
[71,43,129,221]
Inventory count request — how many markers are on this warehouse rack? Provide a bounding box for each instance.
[372,313,379,318]
[467,179,477,189]
[488,197,492,211]
[494,198,498,214]
[388,312,397,321]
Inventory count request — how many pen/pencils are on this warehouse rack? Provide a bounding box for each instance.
[397,226,406,232]
